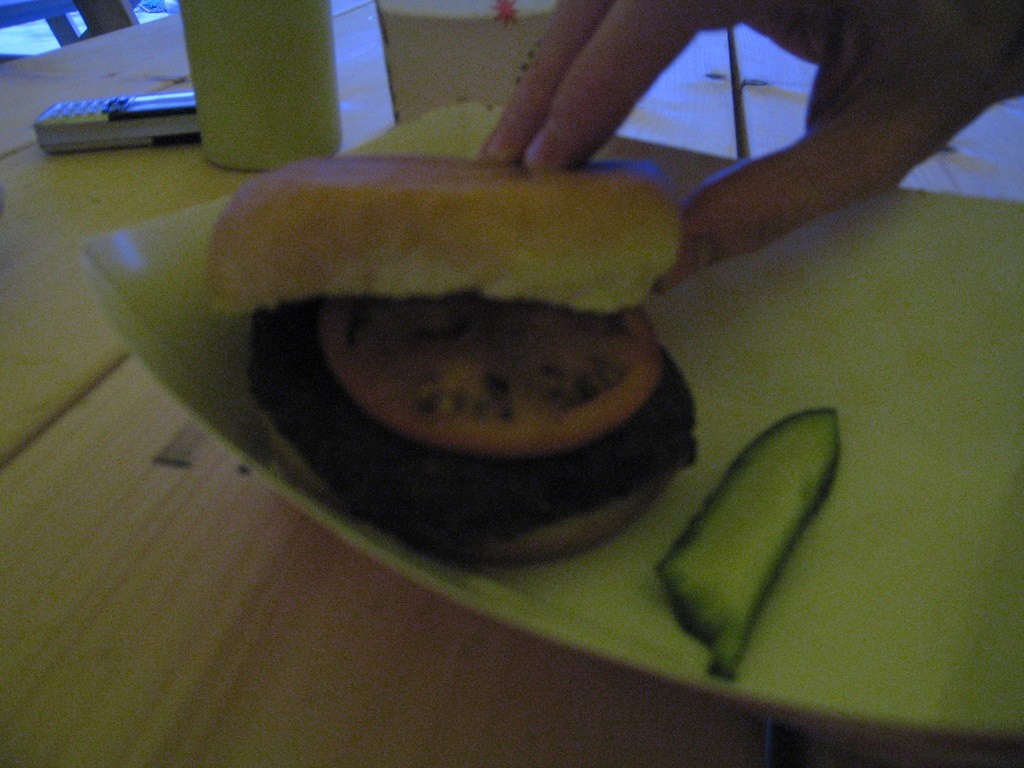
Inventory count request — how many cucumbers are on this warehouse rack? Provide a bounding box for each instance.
[655,406,844,681]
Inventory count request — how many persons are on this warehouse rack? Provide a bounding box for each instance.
[475,0,1024,284]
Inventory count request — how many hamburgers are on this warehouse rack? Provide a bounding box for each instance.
[199,153,702,573]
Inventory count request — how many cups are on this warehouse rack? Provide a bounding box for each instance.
[178,1,341,169]
[375,0,554,126]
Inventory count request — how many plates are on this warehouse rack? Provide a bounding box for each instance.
[66,92,1021,743]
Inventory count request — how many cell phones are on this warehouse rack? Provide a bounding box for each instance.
[35,92,200,157]
